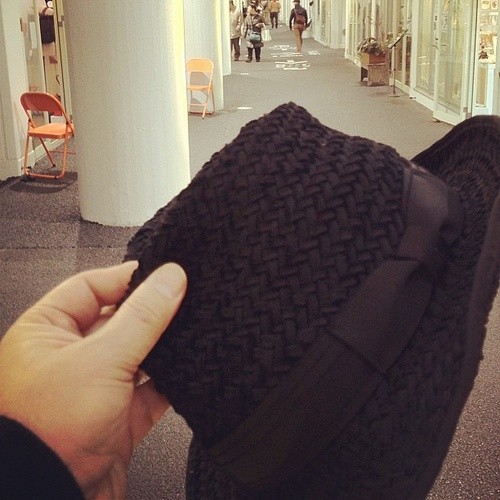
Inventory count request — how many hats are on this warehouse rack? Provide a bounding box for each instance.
[115,101,500,500]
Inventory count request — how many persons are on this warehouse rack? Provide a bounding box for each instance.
[38,0,57,64]
[229,0,308,62]
[0,259,188,500]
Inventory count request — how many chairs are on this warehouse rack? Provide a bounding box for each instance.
[186,57,216,119]
[20,90,74,179]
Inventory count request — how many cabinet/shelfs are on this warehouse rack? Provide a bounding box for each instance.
[358,51,390,87]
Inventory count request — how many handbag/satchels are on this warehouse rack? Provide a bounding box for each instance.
[249,31,261,42]
[295,14,306,24]
